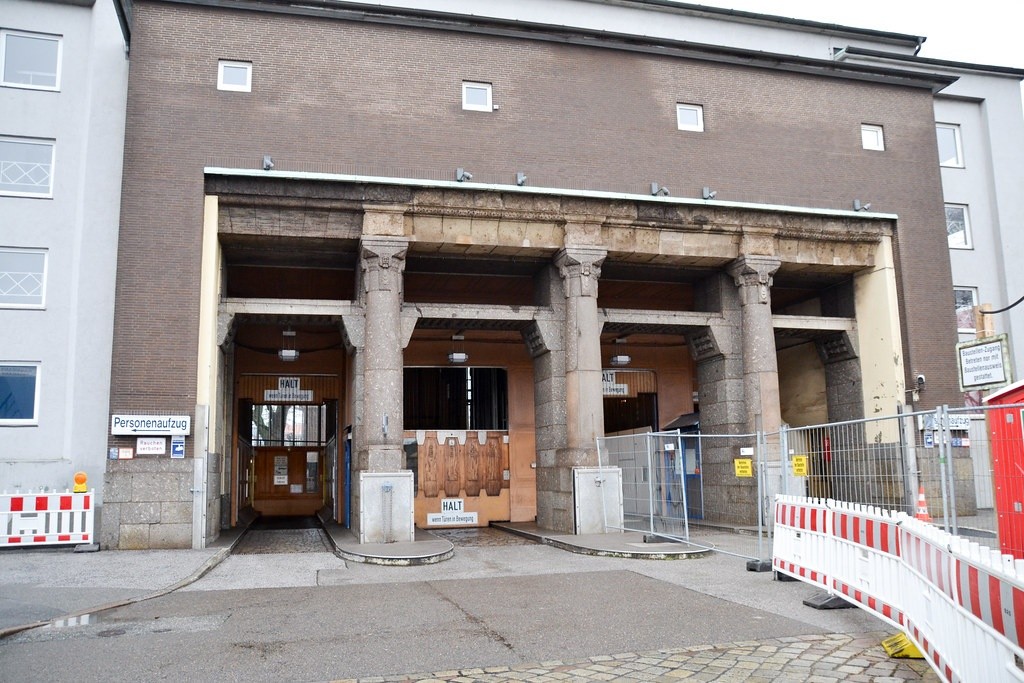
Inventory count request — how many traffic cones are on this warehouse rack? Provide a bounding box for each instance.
[914,486,933,523]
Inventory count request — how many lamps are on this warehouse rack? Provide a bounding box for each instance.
[263,155,274,170]
[610,338,632,367]
[852,199,871,211]
[516,173,527,186]
[456,168,472,182]
[701,187,718,199]
[278,324,299,363]
[650,183,670,196]
[448,335,469,365]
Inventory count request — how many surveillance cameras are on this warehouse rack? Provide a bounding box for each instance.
[917,375,924,384]
